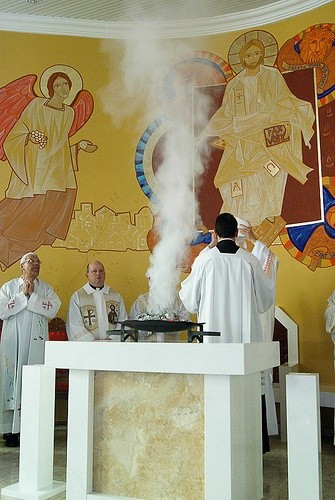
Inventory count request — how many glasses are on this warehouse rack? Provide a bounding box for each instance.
[25,259,42,264]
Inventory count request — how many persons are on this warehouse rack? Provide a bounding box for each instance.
[199,219,280,436]
[178,212,274,454]
[0,252,62,447]
[66,260,130,341]
[129,266,190,341]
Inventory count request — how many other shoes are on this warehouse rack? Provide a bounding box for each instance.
[5,436,20,447]
